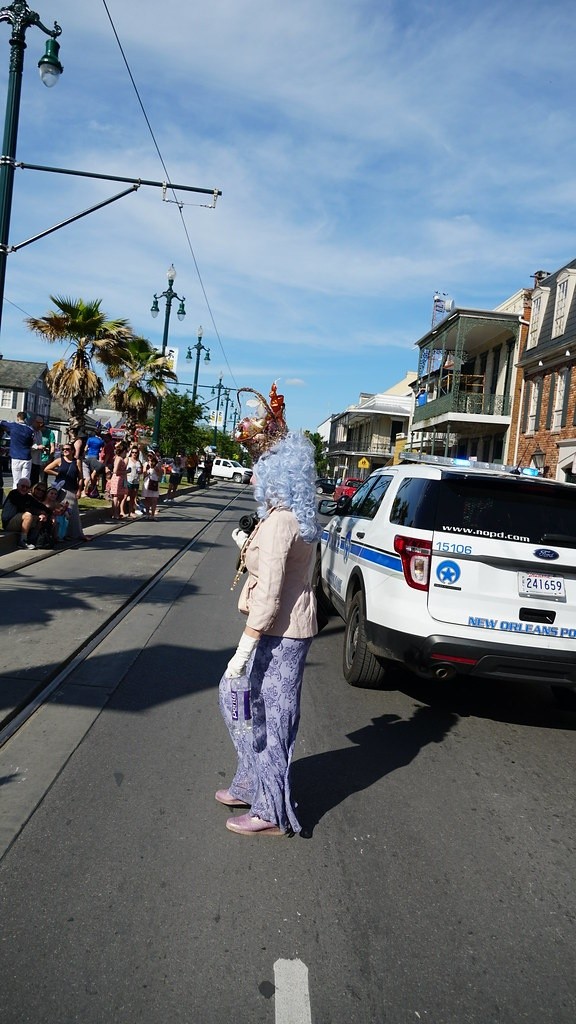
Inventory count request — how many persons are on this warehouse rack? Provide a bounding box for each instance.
[2,479,72,549]
[0,412,114,500]
[110,442,162,520]
[44,444,91,541]
[336,476,343,487]
[215,431,319,835]
[163,450,213,503]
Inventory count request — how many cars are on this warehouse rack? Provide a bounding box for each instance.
[315,478,336,495]
[158,458,174,475]
[198,446,218,462]
[334,478,366,501]
[314,452,576,714]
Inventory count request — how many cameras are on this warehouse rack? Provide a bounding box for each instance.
[239,511,258,538]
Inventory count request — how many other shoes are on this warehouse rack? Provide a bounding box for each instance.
[149,515,154,520]
[142,514,151,519]
[135,510,143,515]
[17,540,36,550]
[163,499,174,502]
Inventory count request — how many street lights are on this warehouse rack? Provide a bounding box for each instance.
[230,409,241,432]
[185,325,211,405]
[0,0,64,328]
[220,388,235,435]
[211,370,229,446]
[149,263,187,442]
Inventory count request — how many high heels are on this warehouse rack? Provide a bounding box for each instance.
[225,813,287,836]
[214,789,247,805]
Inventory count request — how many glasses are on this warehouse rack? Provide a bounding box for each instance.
[131,451,137,454]
[20,484,29,489]
[36,487,45,493]
[147,455,153,458]
[37,422,44,427]
[61,448,70,451]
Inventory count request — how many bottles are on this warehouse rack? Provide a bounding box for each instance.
[230,674,254,735]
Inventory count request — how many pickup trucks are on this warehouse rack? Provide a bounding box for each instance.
[195,458,253,484]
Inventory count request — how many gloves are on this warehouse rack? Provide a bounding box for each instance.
[224,631,260,679]
[232,527,249,550]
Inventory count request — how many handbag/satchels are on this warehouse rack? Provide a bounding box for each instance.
[123,495,135,514]
[147,473,159,491]
[123,479,126,487]
[84,481,93,497]
[90,485,100,498]
